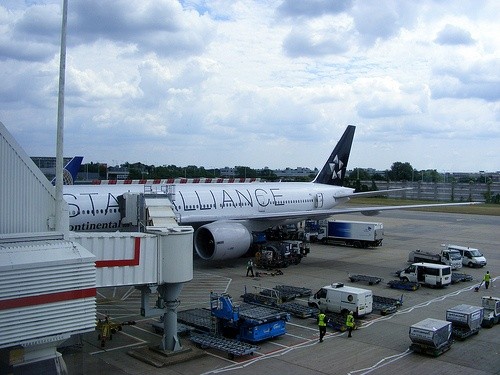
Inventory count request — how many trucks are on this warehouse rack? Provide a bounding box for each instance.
[297,219,318,242]
[254,239,310,267]
[317,220,384,248]
[478,296,500,328]
[407,249,462,272]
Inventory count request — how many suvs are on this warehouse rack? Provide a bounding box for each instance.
[282,224,297,235]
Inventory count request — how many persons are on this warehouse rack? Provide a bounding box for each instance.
[317,309,330,342]
[99,317,109,323]
[346,311,355,338]
[482,271,492,289]
[246,258,255,276]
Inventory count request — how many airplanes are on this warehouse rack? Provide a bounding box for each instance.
[48,157,84,185]
[50,125,485,268]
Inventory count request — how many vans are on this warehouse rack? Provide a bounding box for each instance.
[308,282,373,319]
[399,262,452,289]
[447,245,487,268]
